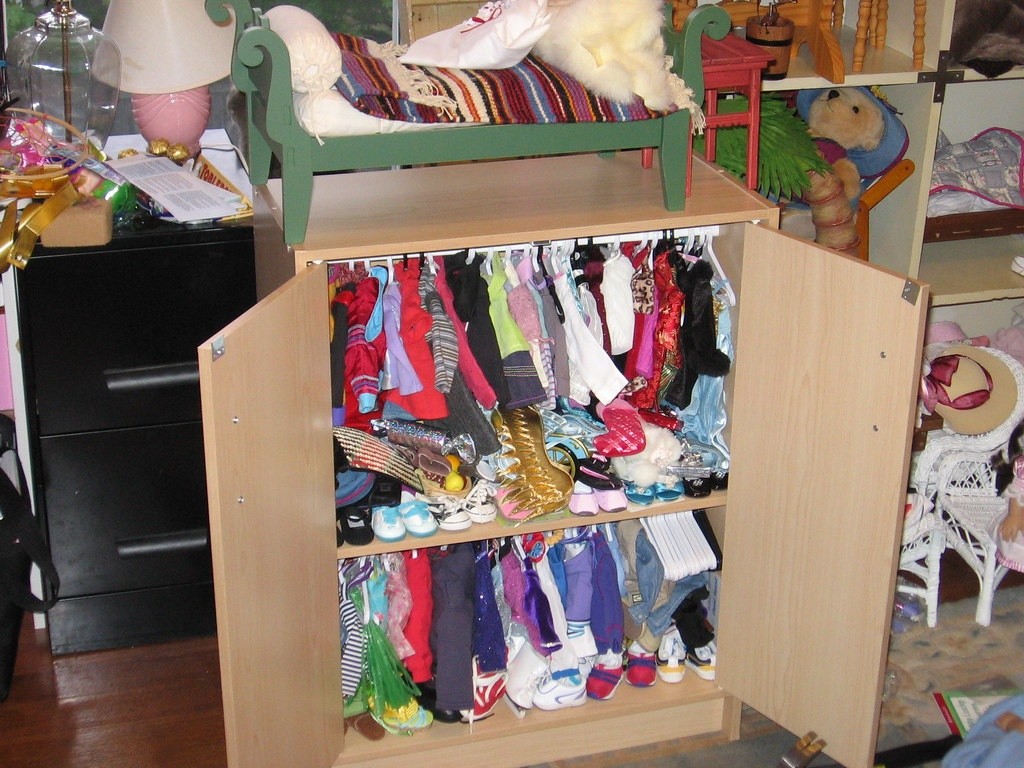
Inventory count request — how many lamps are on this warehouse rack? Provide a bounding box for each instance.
[90,0,237,155]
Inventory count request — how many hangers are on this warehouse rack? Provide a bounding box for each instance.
[348,227,736,309]
[639,509,723,582]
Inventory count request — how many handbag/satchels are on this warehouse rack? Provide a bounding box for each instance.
[0,443,60,703]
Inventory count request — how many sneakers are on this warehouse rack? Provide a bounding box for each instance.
[462,481,498,523]
[684,645,716,681]
[400,501,437,538]
[428,495,472,531]
[626,652,657,687]
[656,651,685,685]
[373,507,406,542]
[586,654,624,700]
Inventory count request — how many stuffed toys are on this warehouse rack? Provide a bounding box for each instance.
[776,87,910,201]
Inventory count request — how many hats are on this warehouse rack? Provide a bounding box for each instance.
[924,345,1019,436]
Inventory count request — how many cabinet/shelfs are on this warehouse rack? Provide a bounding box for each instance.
[14,226,257,658]
[197,0,1023,768]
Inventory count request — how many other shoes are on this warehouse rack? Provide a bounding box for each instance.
[340,507,374,546]
[625,481,654,505]
[346,711,386,741]
[709,471,728,490]
[418,685,462,723]
[655,480,686,501]
[684,477,711,497]
[383,698,435,732]
[343,643,586,741]
[567,485,599,516]
[594,488,628,513]
